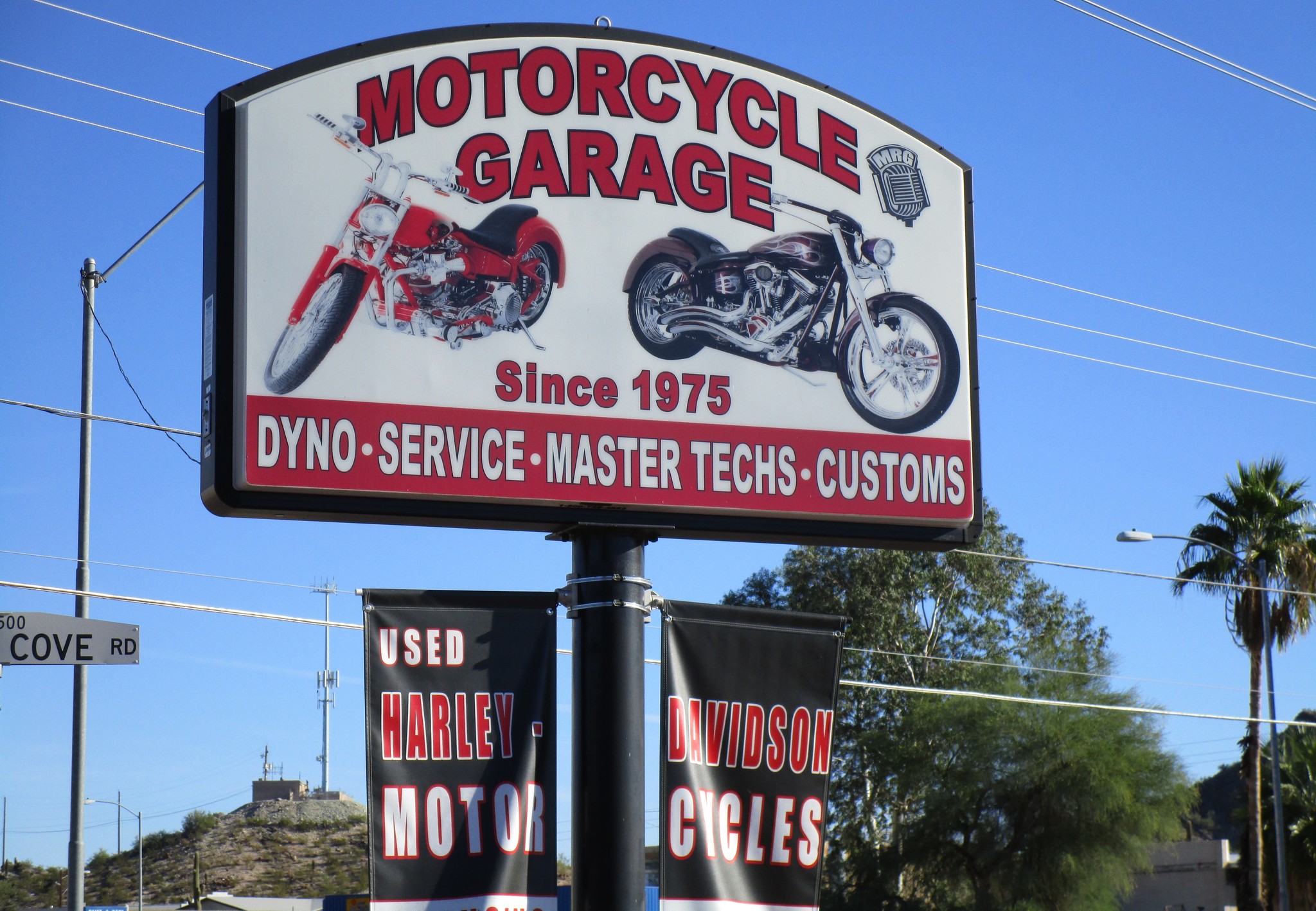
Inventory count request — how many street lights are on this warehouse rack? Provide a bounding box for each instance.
[83,797,143,911]
[60,870,90,907]
[1116,529,1291,911]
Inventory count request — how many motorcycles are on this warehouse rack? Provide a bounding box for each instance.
[622,191,961,434]
[262,113,566,396]
